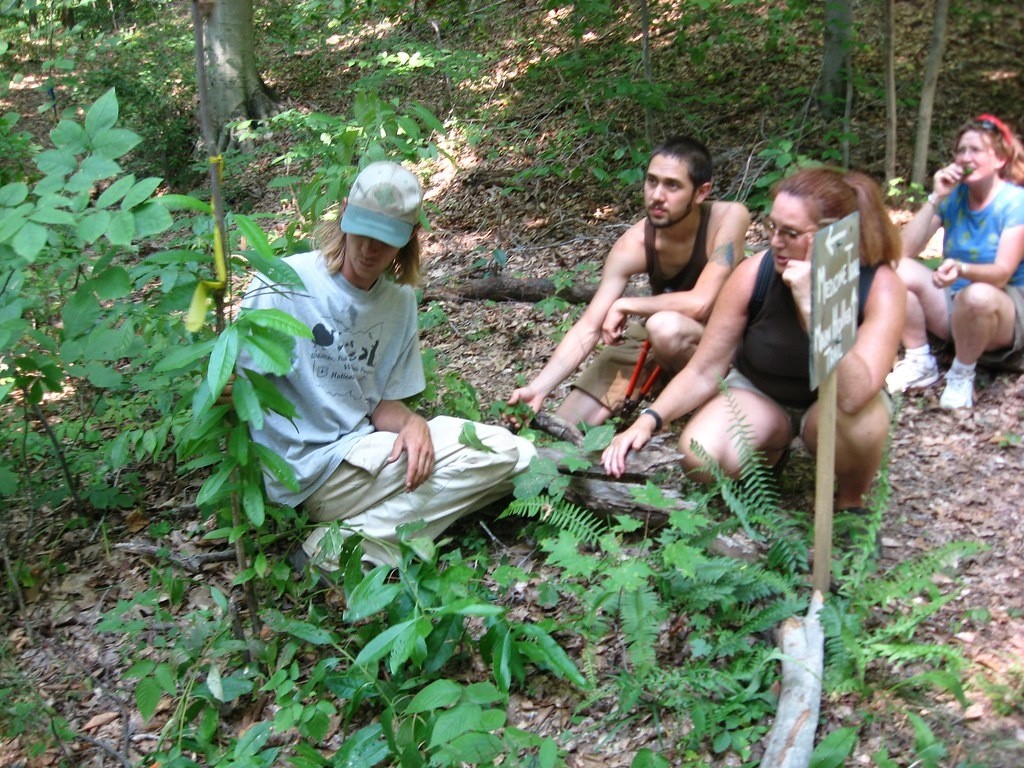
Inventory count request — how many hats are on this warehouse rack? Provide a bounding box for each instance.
[341,161,420,248]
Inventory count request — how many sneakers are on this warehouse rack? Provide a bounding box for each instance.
[885,357,938,395]
[939,372,976,408]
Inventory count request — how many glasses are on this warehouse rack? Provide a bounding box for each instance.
[975,120,1000,133]
[762,219,800,240]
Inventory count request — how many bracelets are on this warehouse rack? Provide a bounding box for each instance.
[641,408,663,433]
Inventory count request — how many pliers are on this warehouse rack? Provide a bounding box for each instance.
[619,340,662,431]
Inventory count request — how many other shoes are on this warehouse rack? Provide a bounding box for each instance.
[761,450,791,480]
[291,549,367,605]
[816,507,881,560]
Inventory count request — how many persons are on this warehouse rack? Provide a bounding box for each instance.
[599,167,908,516]
[511,137,749,439]
[887,115,1024,411]
[237,159,536,580]
